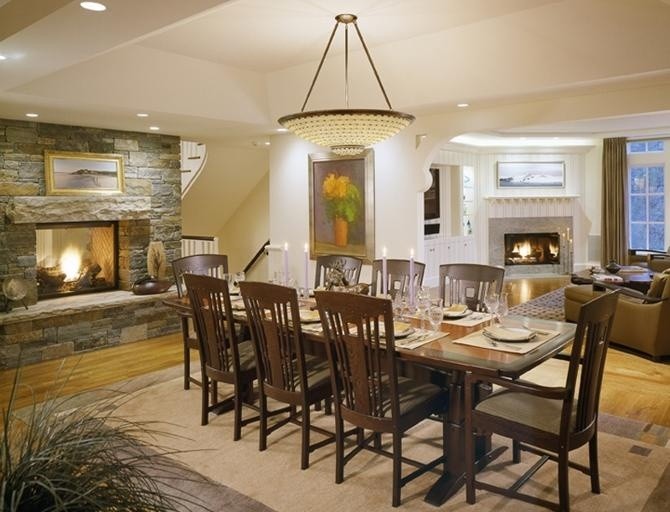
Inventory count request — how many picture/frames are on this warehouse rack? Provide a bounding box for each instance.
[44,149,125,196]
[497,160,566,189]
[308,148,375,264]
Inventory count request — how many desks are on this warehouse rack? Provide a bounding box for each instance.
[572,266,660,294]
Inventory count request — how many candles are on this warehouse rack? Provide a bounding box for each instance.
[409,248,417,313]
[383,246,387,300]
[284,242,288,285]
[566,227,569,244]
[562,233,565,248]
[569,239,573,253]
[303,241,310,299]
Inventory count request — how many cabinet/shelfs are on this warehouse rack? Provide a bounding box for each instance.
[424,166,476,287]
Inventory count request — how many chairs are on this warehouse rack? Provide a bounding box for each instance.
[316,292,450,507]
[372,260,426,297]
[172,254,229,405]
[183,274,297,441]
[438,264,505,322]
[465,287,622,512]
[313,255,362,291]
[239,281,364,467]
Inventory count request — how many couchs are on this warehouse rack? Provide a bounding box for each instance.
[564,249,670,362]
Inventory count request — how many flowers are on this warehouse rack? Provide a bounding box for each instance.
[322,173,361,221]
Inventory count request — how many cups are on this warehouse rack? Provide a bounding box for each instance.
[430,298,443,337]
[485,293,499,326]
[224,273,232,285]
[497,293,509,323]
[416,289,430,335]
[235,271,245,302]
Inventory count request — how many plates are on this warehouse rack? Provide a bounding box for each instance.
[288,309,329,323]
[2,275,28,301]
[483,327,536,342]
[371,323,414,338]
[442,307,473,319]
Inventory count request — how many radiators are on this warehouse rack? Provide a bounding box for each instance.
[586,234,601,265]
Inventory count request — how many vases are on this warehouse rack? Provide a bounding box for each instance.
[335,217,348,248]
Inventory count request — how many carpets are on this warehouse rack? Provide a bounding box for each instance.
[508,284,646,322]
[11,339,670,512]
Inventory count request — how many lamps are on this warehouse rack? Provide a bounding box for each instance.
[278,14,415,155]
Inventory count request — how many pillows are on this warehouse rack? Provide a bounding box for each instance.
[644,273,669,302]
[662,269,670,273]
